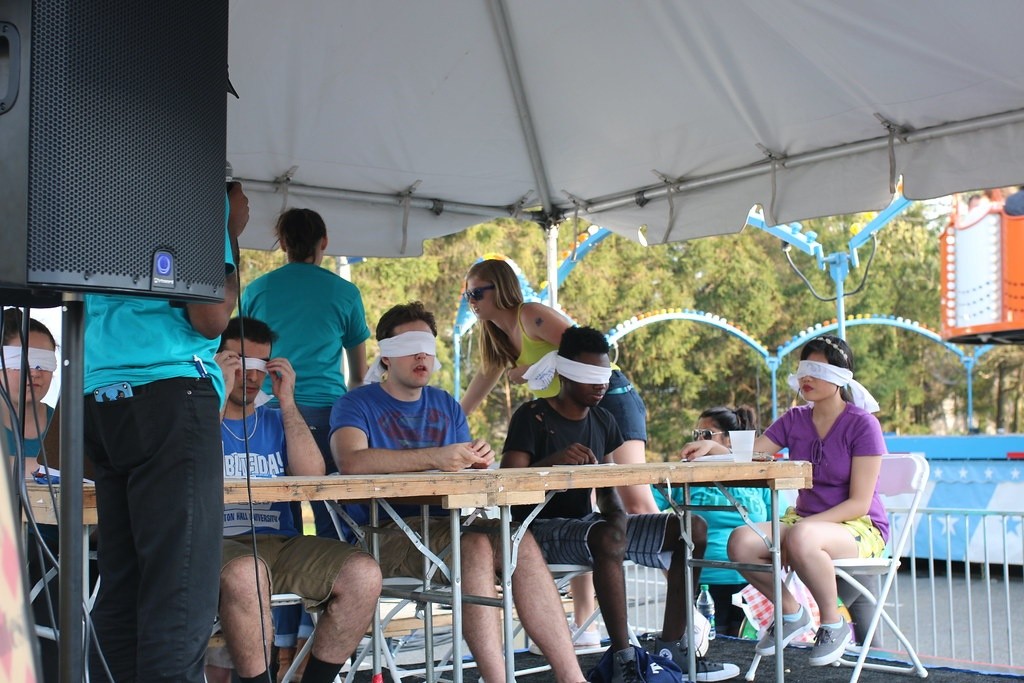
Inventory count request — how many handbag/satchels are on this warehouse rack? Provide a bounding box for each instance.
[731,565,821,646]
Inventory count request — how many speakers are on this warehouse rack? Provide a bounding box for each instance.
[0,1,229,304]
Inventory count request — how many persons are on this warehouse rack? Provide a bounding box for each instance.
[329,300,592,683]
[499,325,740,683]
[458,259,712,657]
[681,336,889,666]
[237,208,371,682]
[219,318,383,683]
[649,405,777,639]
[0,176,249,683]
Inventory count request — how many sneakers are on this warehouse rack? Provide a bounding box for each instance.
[613,647,644,683]
[528,619,600,653]
[654,638,740,681]
[809,614,852,667]
[755,603,815,656]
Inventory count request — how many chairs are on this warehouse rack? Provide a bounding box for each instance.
[213,453,931,683]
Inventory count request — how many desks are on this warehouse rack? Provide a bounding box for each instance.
[17,457,812,683]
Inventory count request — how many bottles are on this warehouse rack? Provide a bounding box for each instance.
[696,583,716,641]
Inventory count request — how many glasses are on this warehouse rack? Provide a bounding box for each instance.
[692,429,730,441]
[464,283,497,302]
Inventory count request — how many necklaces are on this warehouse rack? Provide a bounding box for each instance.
[222,407,258,441]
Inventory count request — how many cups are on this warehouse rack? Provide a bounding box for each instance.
[728,430,756,464]
